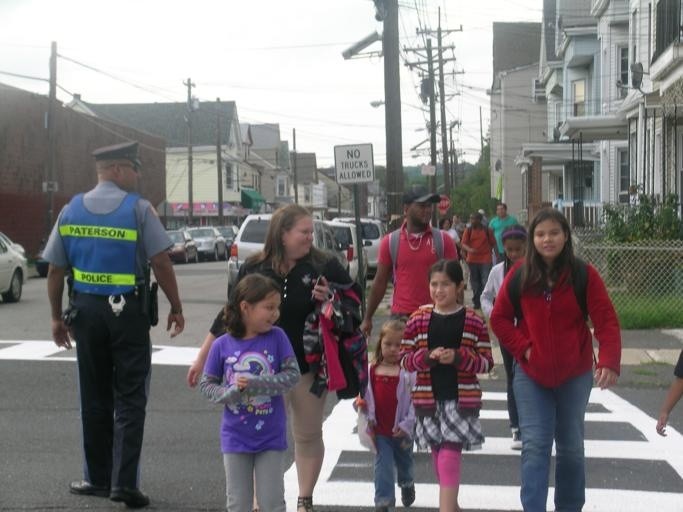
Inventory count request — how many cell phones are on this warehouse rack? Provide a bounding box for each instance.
[309,275,323,302]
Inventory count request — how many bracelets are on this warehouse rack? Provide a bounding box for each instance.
[169,306,183,315]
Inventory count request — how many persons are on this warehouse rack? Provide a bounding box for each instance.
[491,208,621,511]
[655,348,683,438]
[398,258,494,511]
[41,138,185,509]
[200,273,301,512]
[439,203,520,311]
[480,223,530,448]
[188,204,361,511]
[359,183,464,339]
[353,319,417,512]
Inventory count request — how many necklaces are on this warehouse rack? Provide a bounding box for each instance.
[404,228,423,251]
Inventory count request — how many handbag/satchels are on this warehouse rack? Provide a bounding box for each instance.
[336,330,369,399]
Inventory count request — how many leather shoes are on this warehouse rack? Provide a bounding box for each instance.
[109,487,149,508]
[69,479,110,497]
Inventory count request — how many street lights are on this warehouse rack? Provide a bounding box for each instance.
[370,101,437,200]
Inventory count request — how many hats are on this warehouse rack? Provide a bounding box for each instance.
[402,186,441,204]
[92,140,142,167]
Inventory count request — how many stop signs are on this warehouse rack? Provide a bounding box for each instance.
[435,194,451,213]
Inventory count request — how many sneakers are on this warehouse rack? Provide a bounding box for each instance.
[511,429,521,449]
[401,481,415,507]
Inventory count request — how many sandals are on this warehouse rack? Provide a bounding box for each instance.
[298,496,314,512]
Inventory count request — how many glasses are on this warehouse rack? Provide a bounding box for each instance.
[115,162,137,173]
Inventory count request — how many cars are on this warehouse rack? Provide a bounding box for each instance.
[35,235,48,277]
[226,214,350,300]
[0,231,25,259]
[321,220,372,290]
[147,224,238,266]
[0,235,27,303]
[331,216,386,279]
